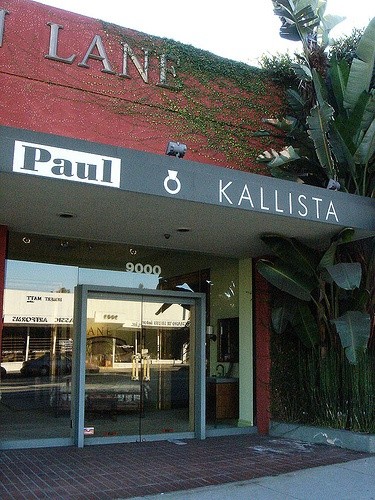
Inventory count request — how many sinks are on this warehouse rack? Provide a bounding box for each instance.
[207,376,239,384]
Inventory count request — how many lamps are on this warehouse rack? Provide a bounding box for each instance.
[165,141,187,158]
[206,326,216,342]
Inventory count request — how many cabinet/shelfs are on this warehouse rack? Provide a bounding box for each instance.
[205,383,239,429]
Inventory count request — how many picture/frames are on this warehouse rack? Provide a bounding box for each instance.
[217,318,239,362]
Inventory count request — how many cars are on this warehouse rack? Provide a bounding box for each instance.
[49,370,149,420]
[0,350,44,380]
[19,351,102,378]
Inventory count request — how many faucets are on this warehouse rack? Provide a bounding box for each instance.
[216,364,224,376]
